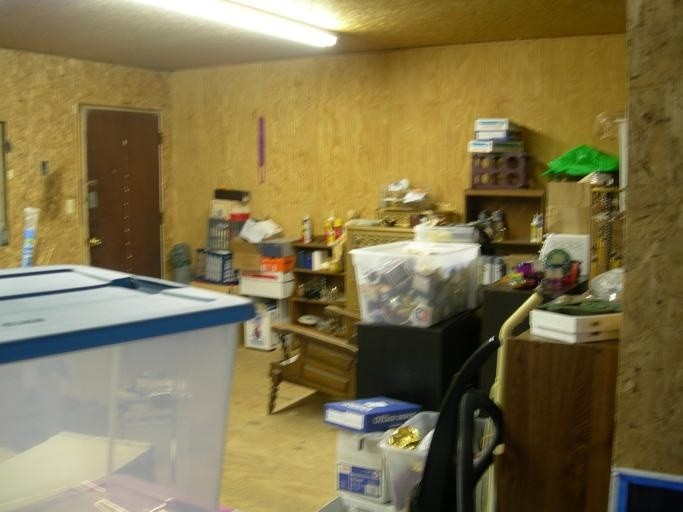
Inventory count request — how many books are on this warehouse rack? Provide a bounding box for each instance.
[193,189,251,284]
[324,396,423,433]
[467,114,525,154]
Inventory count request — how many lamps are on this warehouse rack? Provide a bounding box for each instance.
[131,0,339,50]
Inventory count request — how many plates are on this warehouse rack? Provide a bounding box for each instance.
[297,314,318,326]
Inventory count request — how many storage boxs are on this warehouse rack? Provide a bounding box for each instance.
[0,257,259,512]
[192,198,294,353]
[411,223,487,312]
[346,238,482,331]
[334,410,492,512]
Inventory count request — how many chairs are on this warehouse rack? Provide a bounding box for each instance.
[263,300,361,420]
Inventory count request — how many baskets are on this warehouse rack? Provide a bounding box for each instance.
[471,154,527,188]
[196,218,243,284]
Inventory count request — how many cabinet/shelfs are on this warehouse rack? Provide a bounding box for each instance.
[354,314,484,407]
[493,326,619,512]
[339,221,416,325]
[462,187,544,253]
[479,277,589,378]
[288,239,343,335]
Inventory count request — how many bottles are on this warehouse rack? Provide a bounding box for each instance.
[483,256,503,285]
[530,214,542,244]
[301,215,311,245]
[334,219,342,239]
